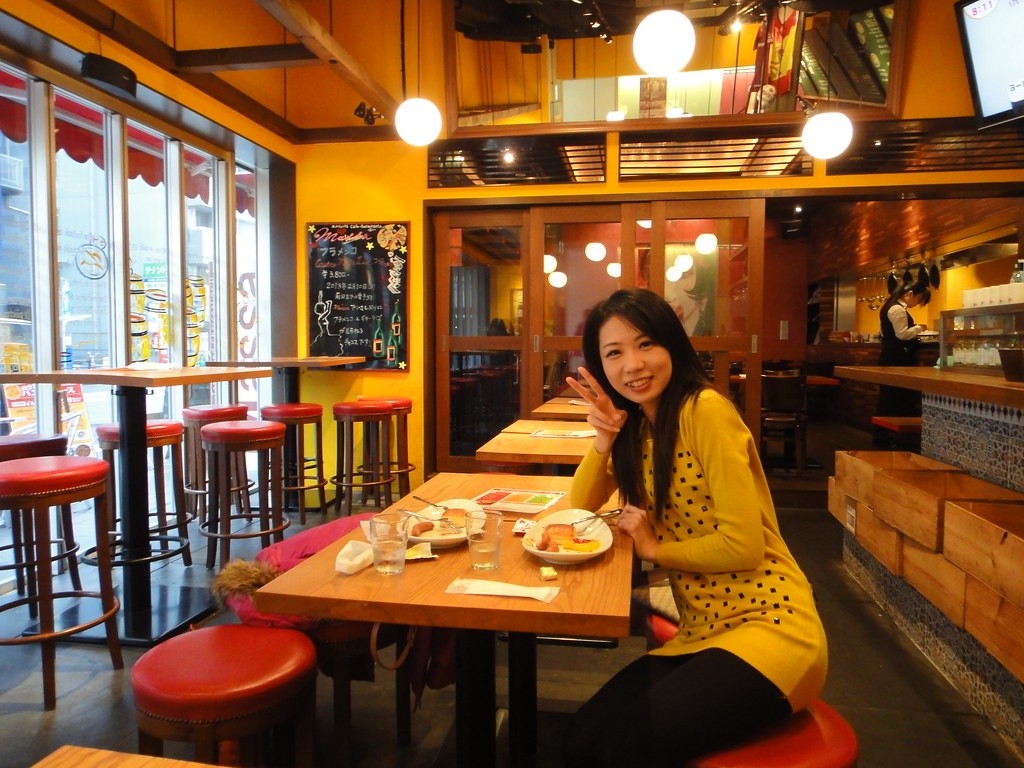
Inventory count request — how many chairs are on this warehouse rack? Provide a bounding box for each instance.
[753,369,811,481]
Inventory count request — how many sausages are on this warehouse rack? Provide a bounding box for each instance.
[536,534,559,553]
[412,521,434,536]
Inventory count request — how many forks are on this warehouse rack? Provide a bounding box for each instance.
[397,509,462,534]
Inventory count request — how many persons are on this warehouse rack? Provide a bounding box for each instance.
[560,289,827,768]
[874,281,931,450]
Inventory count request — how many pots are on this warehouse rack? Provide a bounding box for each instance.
[887,253,944,297]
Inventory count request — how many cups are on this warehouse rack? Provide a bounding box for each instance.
[466,509,503,569]
[370,513,408,574]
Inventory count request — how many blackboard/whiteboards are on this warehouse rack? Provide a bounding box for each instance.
[305,220,411,373]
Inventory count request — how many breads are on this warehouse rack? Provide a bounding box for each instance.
[543,523,600,552]
[441,508,467,528]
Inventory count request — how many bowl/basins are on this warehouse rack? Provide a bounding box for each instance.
[999,347,1024,382]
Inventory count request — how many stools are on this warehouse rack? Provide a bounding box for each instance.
[198,420,291,570]
[260,403,328,526]
[0,456,125,711]
[96,420,193,570]
[0,432,83,618]
[130,623,317,765]
[182,405,252,521]
[697,697,859,768]
[450,359,523,398]
[359,398,415,505]
[330,402,397,517]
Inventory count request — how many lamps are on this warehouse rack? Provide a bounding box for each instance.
[544,252,568,288]
[800,4,852,159]
[606,262,621,279]
[395,1,444,147]
[631,1,697,78]
[354,103,384,125]
[586,240,607,263]
[695,232,718,256]
[666,253,694,283]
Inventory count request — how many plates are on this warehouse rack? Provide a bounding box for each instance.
[398,499,486,549]
[522,508,613,565]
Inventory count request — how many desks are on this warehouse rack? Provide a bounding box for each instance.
[728,372,841,473]
[255,472,636,767]
[871,416,926,437]
[204,356,367,515]
[473,397,622,468]
[0,366,276,645]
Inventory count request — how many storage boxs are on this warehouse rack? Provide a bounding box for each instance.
[825,450,1024,685]
